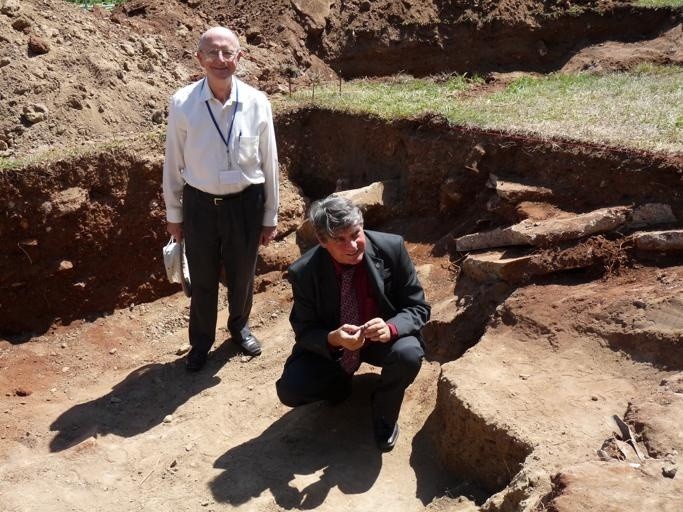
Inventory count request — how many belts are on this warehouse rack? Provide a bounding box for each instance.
[183,182,265,207]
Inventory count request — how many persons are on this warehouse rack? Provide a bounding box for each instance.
[272,192,433,453]
[161,24,281,371]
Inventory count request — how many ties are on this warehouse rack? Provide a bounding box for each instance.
[338,266,367,375]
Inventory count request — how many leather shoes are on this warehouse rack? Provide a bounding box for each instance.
[373,413,399,451]
[186,347,209,370]
[236,333,262,356]
